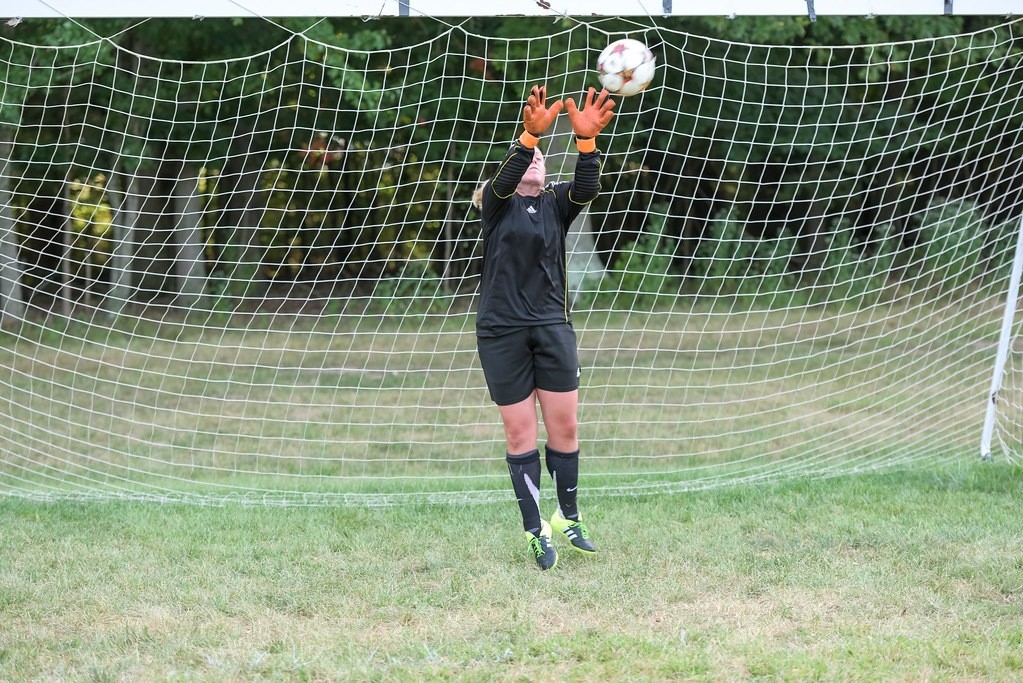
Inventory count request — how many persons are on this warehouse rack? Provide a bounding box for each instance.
[469,84,615,572]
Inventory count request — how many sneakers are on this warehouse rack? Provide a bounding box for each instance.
[525,518,558,572]
[550,510,598,554]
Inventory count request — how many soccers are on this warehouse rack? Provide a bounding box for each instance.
[595,38,656,98]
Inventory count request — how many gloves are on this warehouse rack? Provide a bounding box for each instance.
[523,85,564,137]
[565,87,616,140]
